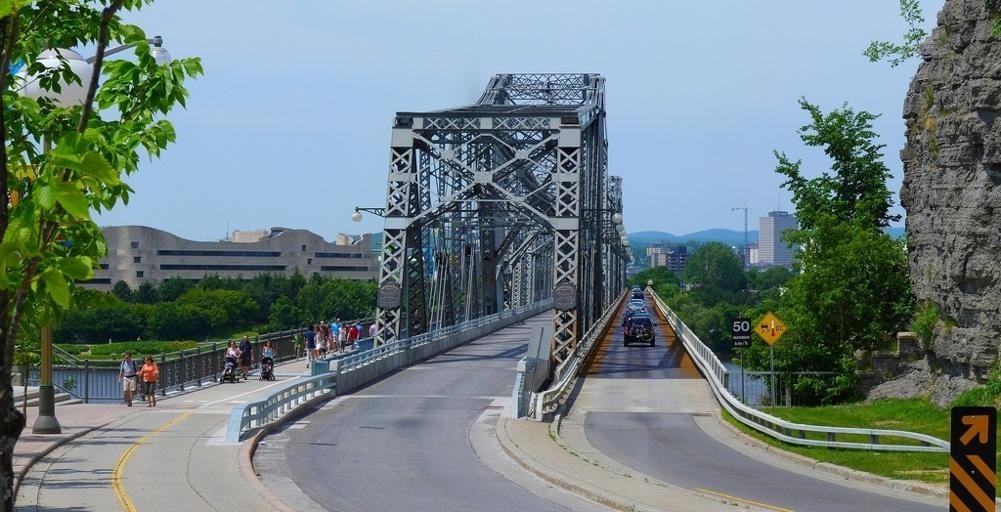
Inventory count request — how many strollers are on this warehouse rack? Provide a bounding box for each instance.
[220,352,250,384]
[259,356,275,381]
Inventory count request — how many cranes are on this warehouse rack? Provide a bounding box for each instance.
[731,207,752,245]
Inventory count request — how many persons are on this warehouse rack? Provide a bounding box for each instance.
[263,339,277,373]
[240,335,253,379]
[369,321,376,336]
[118,352,138,406]
[265,360,272,371]
[138,356,159,407]
[303,318,364,368]
[225,360,235,373]
[227,340,240,368]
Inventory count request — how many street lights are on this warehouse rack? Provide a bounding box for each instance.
[8,35,171,434]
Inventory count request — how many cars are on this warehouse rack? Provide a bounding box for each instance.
[621,284,658,347]
